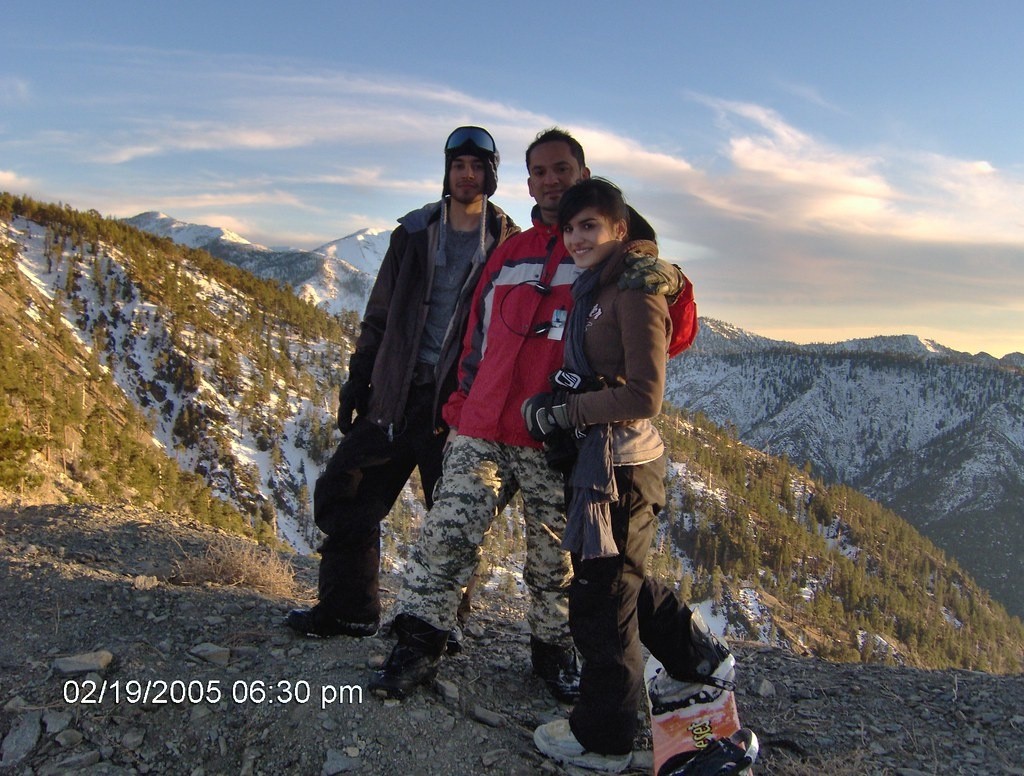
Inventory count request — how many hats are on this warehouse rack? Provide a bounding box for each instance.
[435,146,500,267]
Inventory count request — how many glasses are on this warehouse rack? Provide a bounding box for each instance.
[445,126,495,153]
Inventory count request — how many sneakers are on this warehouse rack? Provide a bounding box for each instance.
[651,659,736,703]
[287,595,380,638]
[534,720,632,773]
[446,613,466,648]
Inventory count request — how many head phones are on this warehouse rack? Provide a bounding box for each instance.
[499,280,552,338]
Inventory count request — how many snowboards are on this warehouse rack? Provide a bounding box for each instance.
[643,636,759,776]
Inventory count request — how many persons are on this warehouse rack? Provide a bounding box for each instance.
[521,176,736,773]
[365,130,699,705]
[287,126,521,640]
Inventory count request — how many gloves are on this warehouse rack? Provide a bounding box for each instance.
[338,354,376,435]
[545,368,602,471]
[520,392,573,442]
[617,252,685,300]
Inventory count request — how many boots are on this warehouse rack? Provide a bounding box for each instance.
[369,614,451,700]
[530,635,582,705]
